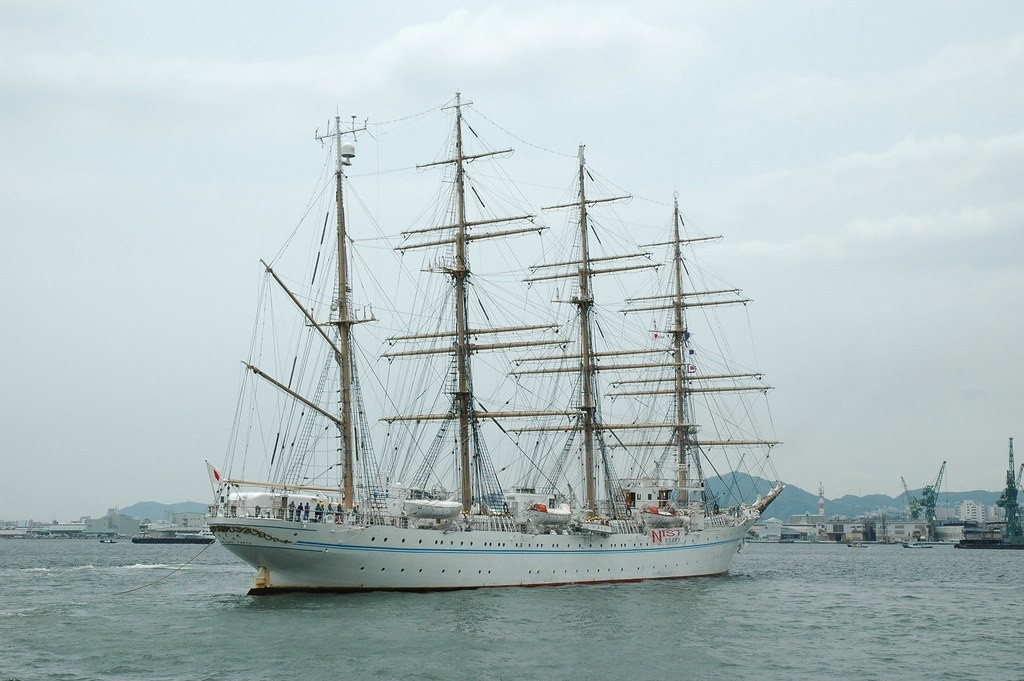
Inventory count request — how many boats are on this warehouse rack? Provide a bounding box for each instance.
[902,542,933,548]
[847,542,868,547]
[132,518,215,544]
[100,538,117,543]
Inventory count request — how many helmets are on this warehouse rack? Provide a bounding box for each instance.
[317,502,320,504]
[328,502,332,506]
[321,503,324,506]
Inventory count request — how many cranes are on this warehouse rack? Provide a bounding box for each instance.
[901,475,922,515]
[920,461,947,506]
[996,438,1024,508]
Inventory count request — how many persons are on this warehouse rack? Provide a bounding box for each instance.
[288,501,298,522]
[296,503,304,523]
[327,502,336,513]
[352,505,361,526]
[304,503,310,520]
[335,503,344,522]
[314,503,324,523]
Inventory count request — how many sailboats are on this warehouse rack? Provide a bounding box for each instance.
[204,88,787,592]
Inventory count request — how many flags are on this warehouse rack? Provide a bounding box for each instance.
[205,461,223,485]
